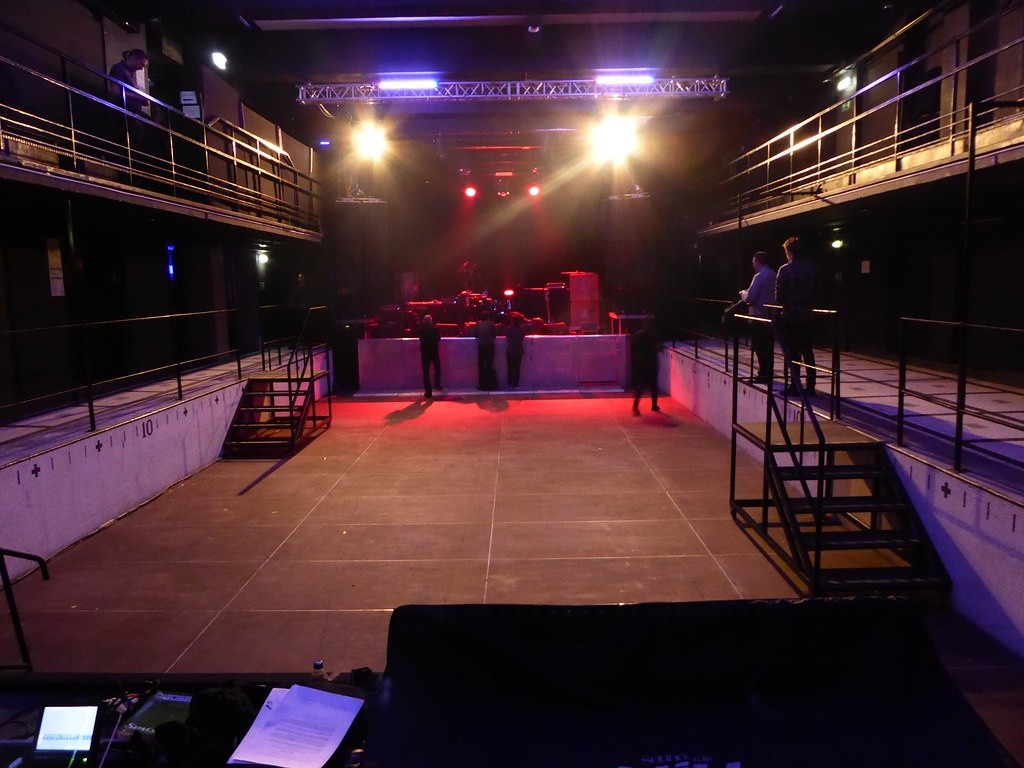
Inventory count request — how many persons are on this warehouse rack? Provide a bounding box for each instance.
[419,314,443,398]
[474,311,496,391]
[631,313,663,415]
[110,49,151,187]
[774,237,817,395]
[505,315,525,391]
[739,251,777,384]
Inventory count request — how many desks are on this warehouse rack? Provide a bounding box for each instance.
[608,312,654,333]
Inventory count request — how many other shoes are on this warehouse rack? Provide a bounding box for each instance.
[752,371,774,383]
[632,407,640,416]
[804,384,816,396]
[651,403,660,411]
[781,382,804,397]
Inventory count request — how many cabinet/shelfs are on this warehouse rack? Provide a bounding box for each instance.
[559,268,604,335]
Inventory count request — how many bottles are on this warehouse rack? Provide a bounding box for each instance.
[310,660,328,692]
